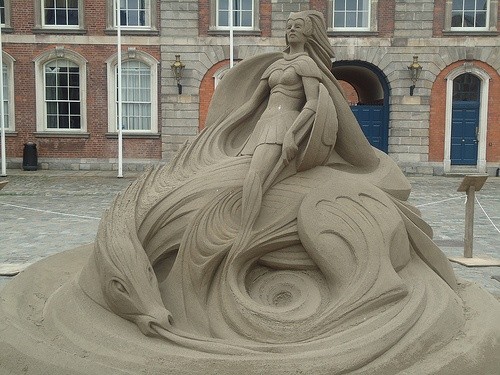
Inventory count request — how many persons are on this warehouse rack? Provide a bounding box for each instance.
[210,9,337,235]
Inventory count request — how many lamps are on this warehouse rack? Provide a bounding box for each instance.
[170,54,185,85]
[406,55,422,84]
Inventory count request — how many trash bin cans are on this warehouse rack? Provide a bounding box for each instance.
[22,142,38,171]
